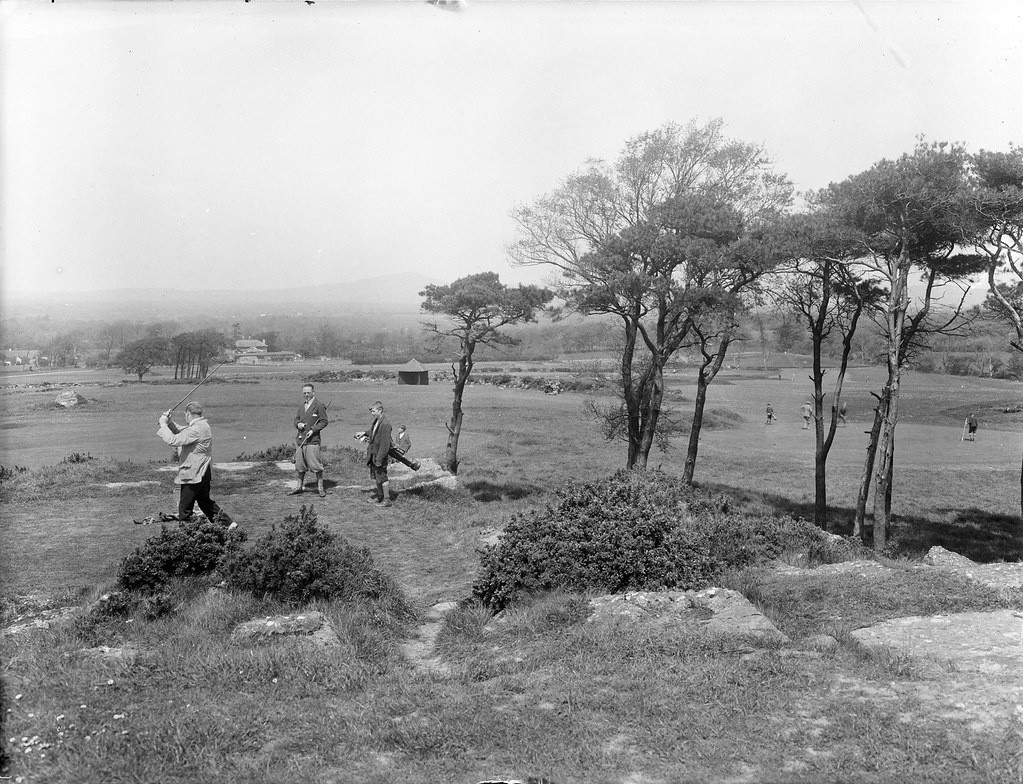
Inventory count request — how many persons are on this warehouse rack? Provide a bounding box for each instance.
[287,383,328,498]
[964,413,978,441]
[394,424,411,463]
[766,404,774,424]
[801,401,813,430]
[837,406,848,424]
[354,401,393,508]
[156,401,239,532]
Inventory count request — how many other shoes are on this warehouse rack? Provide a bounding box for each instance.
[286,487,303,496]
[317,486,326,497]
[367,495,384,503]
[226,521,239,534]
[374,499,392,507]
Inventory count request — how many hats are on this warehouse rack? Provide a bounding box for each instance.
[805,401,810,404]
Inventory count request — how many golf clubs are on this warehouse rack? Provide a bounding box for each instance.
[355,435,370,442]
[155,359,235,426]
[291,400,333,464]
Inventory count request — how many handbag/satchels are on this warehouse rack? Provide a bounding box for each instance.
[771,413,777,420]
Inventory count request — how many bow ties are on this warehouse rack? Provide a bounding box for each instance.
[304,400,309,404]
